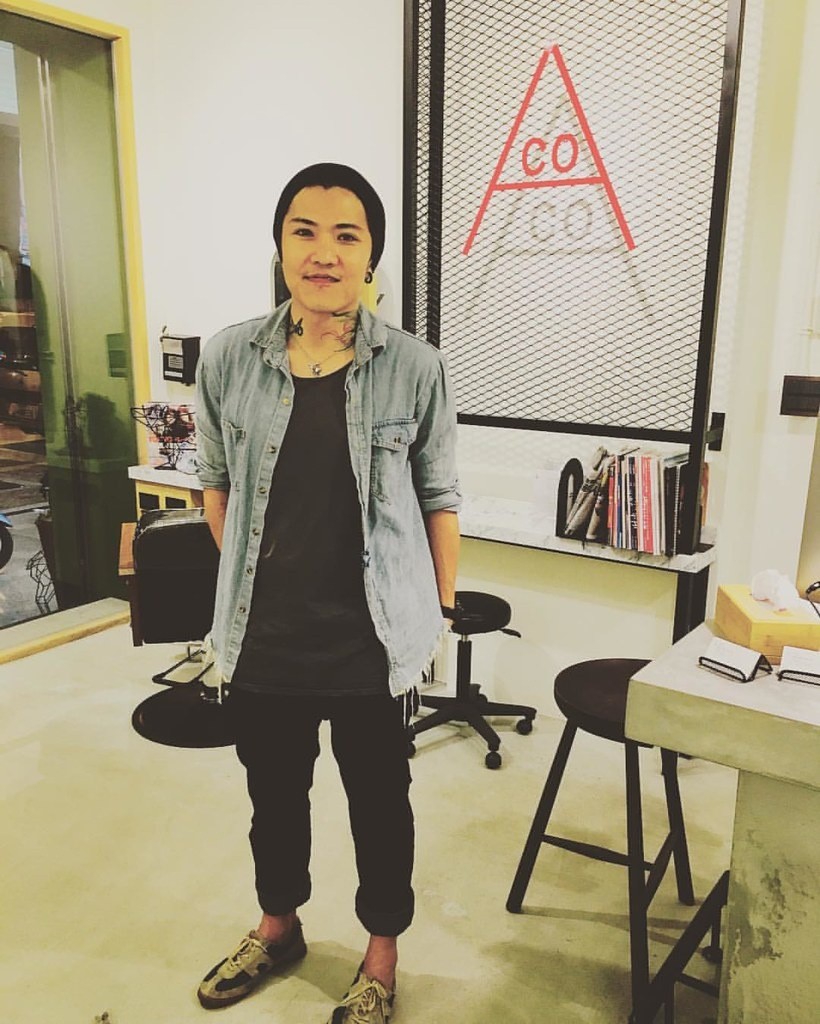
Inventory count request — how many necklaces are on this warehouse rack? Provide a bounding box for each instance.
[292,335,342,377]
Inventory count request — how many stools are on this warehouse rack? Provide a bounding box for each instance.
[505,658,695,1005]
[407,591,536,770]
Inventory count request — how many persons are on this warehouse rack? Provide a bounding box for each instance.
[198,163,464,1023]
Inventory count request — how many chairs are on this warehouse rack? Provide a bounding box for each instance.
[125,507,240,748]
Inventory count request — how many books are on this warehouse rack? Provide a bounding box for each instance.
[565,447,707,559]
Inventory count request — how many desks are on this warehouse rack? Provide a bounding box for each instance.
[128,463,715,645]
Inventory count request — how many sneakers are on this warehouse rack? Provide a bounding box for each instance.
[197,916,307,1010]
[325,960,396,1024]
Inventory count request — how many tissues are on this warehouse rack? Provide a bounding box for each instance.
[715,567,819,664]
